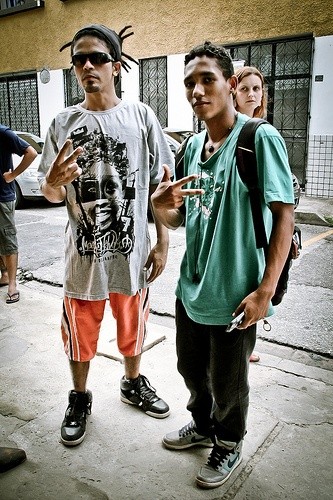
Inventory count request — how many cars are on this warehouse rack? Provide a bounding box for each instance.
[163,127,303,215]
[8,131,57,208]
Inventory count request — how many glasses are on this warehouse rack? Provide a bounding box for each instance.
[71,52,114,67]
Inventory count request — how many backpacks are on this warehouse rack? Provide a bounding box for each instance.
[174,116,302,307]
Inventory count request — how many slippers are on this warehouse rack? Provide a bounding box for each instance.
[5,290,19,303]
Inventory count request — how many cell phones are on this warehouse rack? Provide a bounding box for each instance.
[225,311,245,332]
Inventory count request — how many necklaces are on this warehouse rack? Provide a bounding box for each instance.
[205,112,239,153]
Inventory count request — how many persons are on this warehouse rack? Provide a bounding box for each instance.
[37,24,176,447]
[233,67,300,363]
[151,41,296,488]
[0,124,37,304]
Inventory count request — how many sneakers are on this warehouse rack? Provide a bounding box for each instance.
[119,374,169,419]
[195,435,243,488]
[60,390,92,448]
[162,420,213,449]
